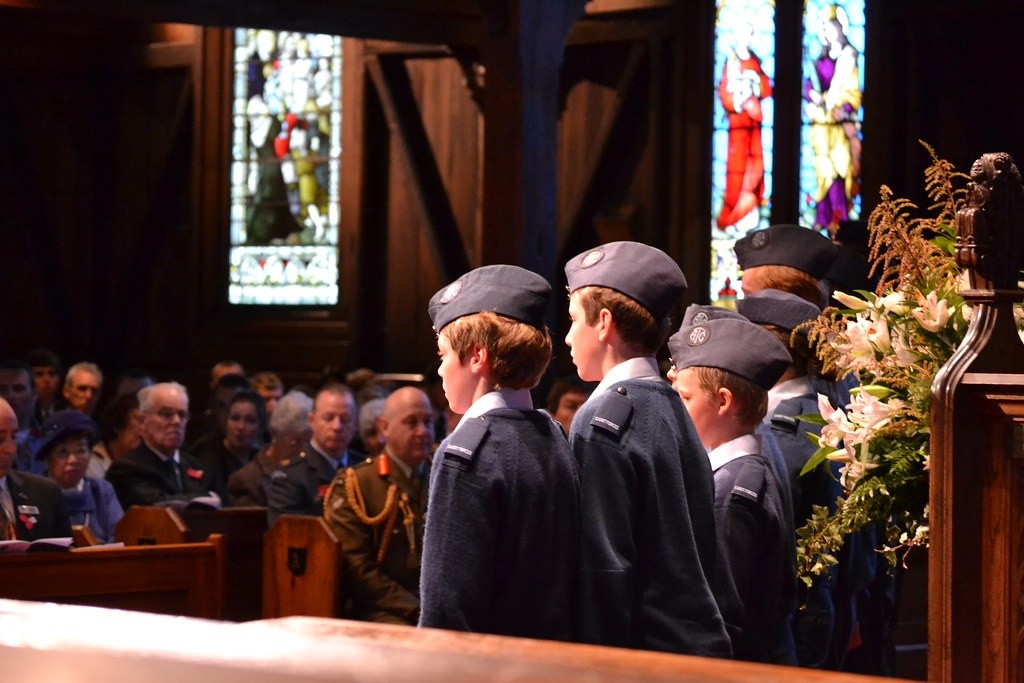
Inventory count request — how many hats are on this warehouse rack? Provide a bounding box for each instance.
[826,216,872,249]
[668,318,794,390]
[30,409,100,460]
[427,264,554,336]
[732,224,837,282]
[731,288,821,334]
[678,303,751,333]
[564,241,692,332]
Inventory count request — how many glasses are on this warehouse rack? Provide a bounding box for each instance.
[53,446,91,458]
[143,409,189,424]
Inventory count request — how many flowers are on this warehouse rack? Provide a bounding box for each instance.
[789,139,1024,591]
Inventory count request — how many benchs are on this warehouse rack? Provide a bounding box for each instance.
[261,515,343,619]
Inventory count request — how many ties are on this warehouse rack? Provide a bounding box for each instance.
[337,460,347,469]
[412,471,420,507]
[167,459,181,494]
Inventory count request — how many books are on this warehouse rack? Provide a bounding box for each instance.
[0,535,75,554]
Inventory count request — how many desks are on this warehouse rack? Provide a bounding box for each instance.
[115,506,269,620]
[0,533,223,619]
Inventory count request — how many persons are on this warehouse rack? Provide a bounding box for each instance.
[563,241,748,662]
[0,350,595,630]
[663,219,898,678]
[417,265,586,646]
